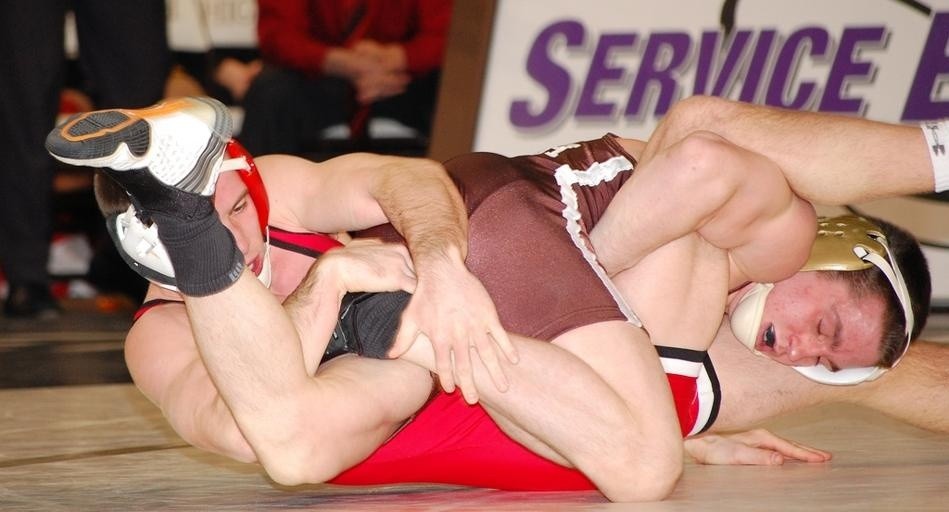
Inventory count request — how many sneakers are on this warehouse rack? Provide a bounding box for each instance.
[45,96,235,197]
[319,290,374,367]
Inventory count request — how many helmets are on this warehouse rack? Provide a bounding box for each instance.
[107,133,268,296]
[730,213,908,387]
